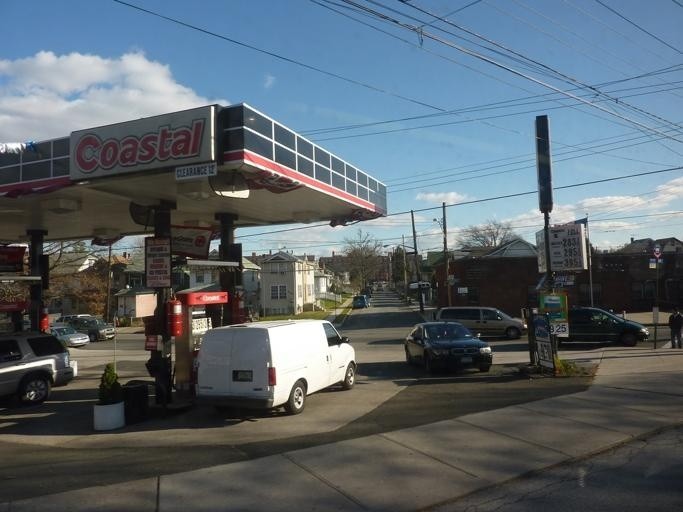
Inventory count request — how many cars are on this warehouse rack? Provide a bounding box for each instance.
[405,322,492,371]
[50,314,114,349]
[436,306,529,339]
[0,332,77,406]
[352,283,384,309]
[555,306,648,346]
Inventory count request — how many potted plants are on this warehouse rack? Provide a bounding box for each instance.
[93,363,127,432]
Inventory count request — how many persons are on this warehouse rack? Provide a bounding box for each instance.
[668,305,683,348]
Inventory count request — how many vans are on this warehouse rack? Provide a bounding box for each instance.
[193,319,357,415]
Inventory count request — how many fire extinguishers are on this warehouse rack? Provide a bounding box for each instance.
[231,292,245,324]
[166,293,183,336]
[40,301,49,330]
